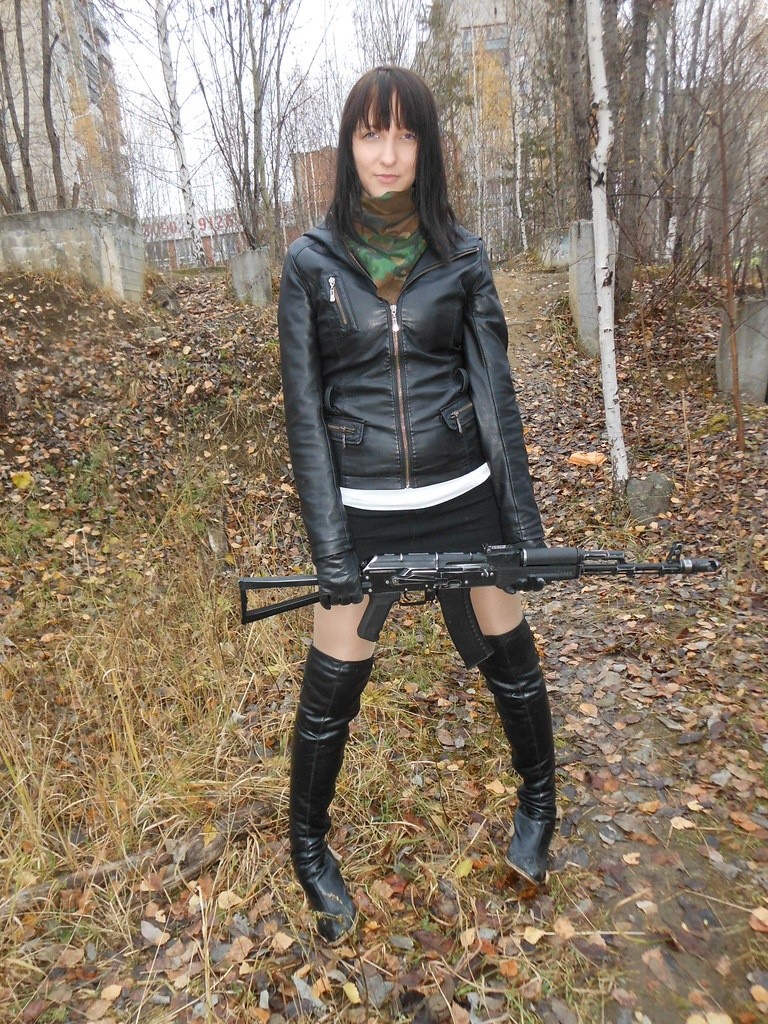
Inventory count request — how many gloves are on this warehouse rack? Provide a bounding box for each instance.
[316,550,364,609]
[498,538,552,594]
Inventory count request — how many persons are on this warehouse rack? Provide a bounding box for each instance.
[278,66,555,947]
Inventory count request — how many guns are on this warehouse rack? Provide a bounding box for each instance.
[235,534,723,631]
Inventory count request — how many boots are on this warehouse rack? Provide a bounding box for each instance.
[475,618,557,887]
[289,641,375,947]
